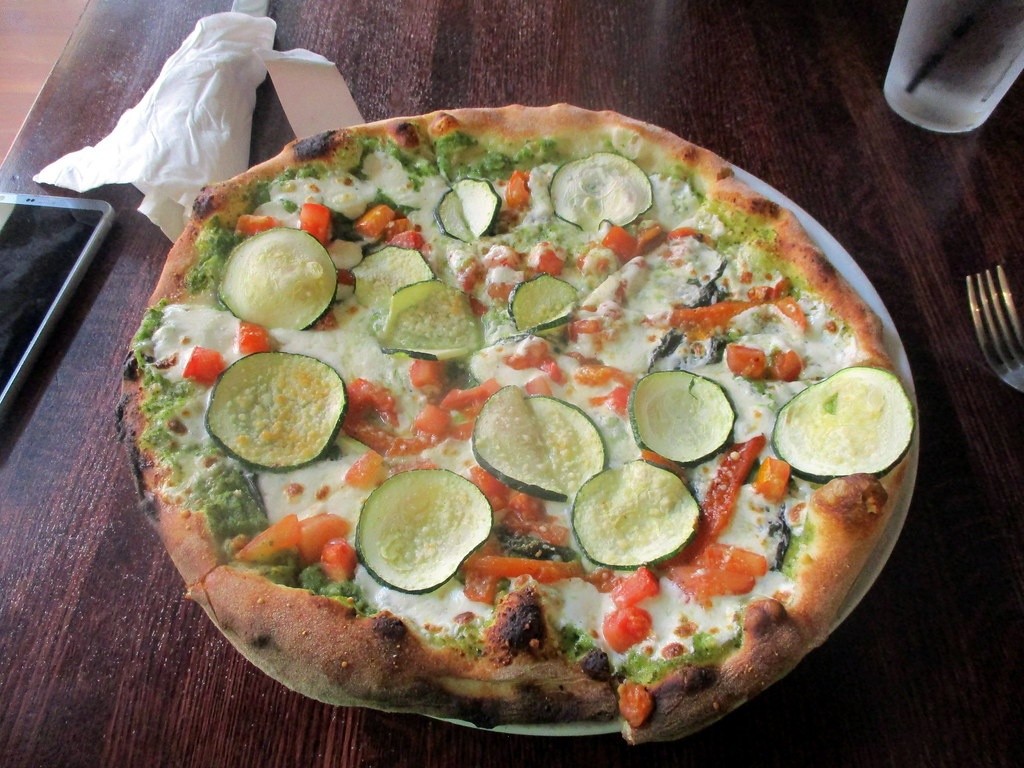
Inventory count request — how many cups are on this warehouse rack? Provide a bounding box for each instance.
[882,0,1024,134]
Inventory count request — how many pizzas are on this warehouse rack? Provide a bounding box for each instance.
[113,101,916,743]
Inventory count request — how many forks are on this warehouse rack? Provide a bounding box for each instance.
[966,266,1024,391]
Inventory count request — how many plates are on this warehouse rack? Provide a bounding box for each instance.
[427,165,921,737]
